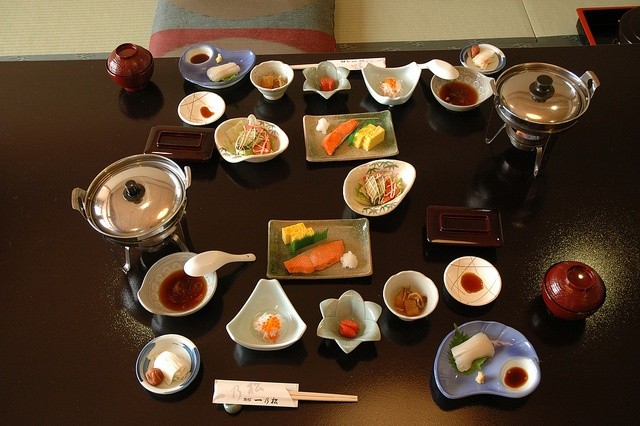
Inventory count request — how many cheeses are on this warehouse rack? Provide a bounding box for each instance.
[362,125,385,151]
[353,123,376,149]
[282,222,306,245]
[290,228,315,242]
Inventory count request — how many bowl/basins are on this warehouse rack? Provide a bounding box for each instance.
[213,118,289,164]
[443,256,502,308]
[136,251,218,320]
[249,61,295,101]
[383,269,439,323]
[343,159,417,216]
[301,61,352,100]
[315,290,382,355]
[225,278,307,354]
[430,66,495,112]
[176,91,226,126]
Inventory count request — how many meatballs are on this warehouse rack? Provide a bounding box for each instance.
[470,45,481,58]
[146,368,163,387]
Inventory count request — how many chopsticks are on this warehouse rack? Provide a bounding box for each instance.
[288,64,318,69]
[289,391,359,401]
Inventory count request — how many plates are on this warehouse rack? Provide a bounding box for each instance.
[302,110,399,163]
[459,43,506,75]
[361,62,422,107]
[266,218,374,280]
[178,43,256,89]
[434,321,541,399]
[135,334,200,397]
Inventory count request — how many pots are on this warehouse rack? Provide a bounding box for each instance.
[70,153,191,255]
[489,63,601,135]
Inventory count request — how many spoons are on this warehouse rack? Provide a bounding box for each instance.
[417,58,459,80]
[183,248,256,276]
